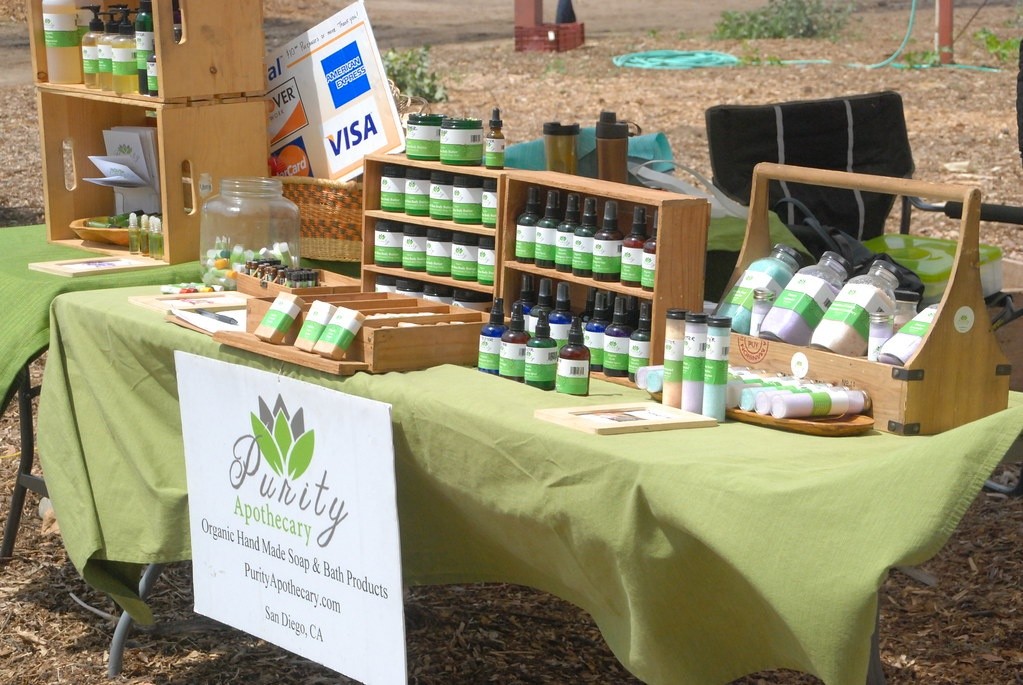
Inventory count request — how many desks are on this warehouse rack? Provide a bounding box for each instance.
[0,224,350,567]
[35,280,1023,685]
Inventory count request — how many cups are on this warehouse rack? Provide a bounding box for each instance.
[543,122,579,176]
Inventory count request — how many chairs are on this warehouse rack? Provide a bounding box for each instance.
[705,87,1023,245]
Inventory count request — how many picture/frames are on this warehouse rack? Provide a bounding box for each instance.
[533,401,718,436]
[28,255,171,277]
[128,290,258,316]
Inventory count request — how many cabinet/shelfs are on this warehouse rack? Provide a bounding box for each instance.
[364,152,712,397]
[26,0,272,267]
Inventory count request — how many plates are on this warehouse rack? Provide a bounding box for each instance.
[68,216,129,246]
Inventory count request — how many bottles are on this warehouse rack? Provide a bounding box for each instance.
[42,0,103,85]
[485,107,505,170]
[199,177,302,290]
[478,187,657,398]
[596,111,642,184]
[134,0,182,96]
[712,243,940,367]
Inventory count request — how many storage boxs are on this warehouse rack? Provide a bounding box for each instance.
[713,162,1012,438]
[236,266,513,376]
